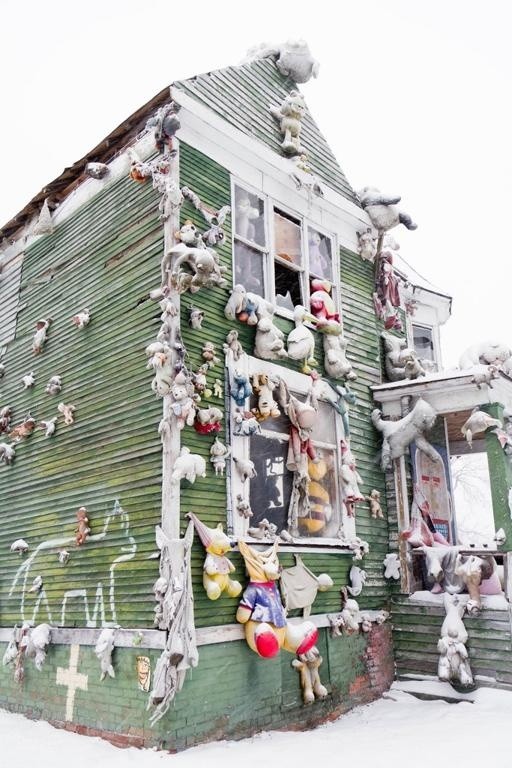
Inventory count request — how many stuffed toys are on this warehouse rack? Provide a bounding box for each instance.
[0,405,12,434]
[28,576,45,593]
[39,416,58,437]
[58,402,76,423]
[10,420,36,440]
[10,539,30,555]
[76,506,91,545]
[58,550,72,561]
[23,372,37,387]
[75,307,92,326]
[46,376,63,392]
[131,105,511,708]
[94,628,116,682]
[269,90,311,155]
[1,440,19,464]
[33,317,52,354]
